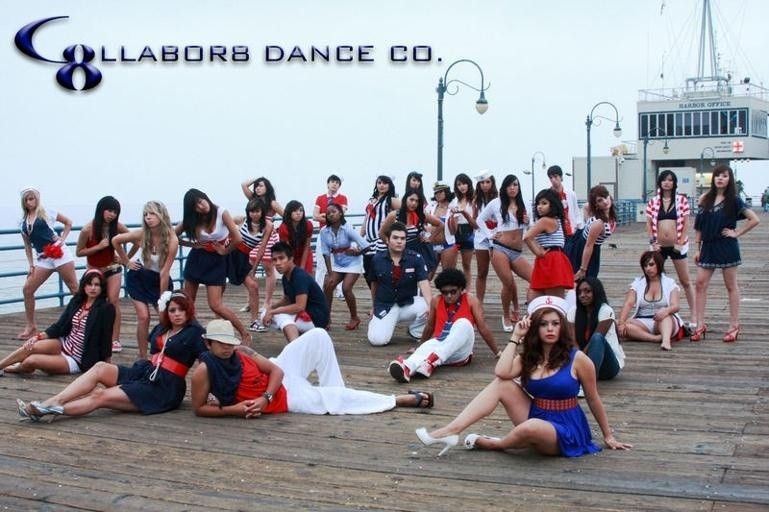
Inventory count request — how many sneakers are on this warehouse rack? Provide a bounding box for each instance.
[415,361,434,378]
[389,360,410,383]
[249,320,269,332]
[112,341,122,352]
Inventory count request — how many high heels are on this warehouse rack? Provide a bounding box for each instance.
[16,398,41,424]
[18,324,37,340]
[30,401,64,424]
[464,433,501,450]
[501,316,513,332]
[690,323,708,341]
[722,323,740,343]
[240,303,251,312]
[511,310,520,321]
[344,319,361,330]
[415,427,459,457]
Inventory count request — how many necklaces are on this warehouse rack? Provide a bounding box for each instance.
[175,326,181,331]
[662,198,671,203]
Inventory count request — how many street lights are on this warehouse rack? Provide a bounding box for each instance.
[434,57,492,186]
[698,147,715,195]
[641,129,669,202]
[583,98,623,200]
[522,150,548,206]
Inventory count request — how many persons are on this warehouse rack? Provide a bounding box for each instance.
[387,268,503,384]
[643,169,697,328]
[261,240,330,344]
[17,186,80,341]
[221,197,274,333]
[75,195,141,354]
[475,174,534,333]
[377,188,446,274]
[569,184,617,284]
[566,275,626,398]
[546,165,584,255]
[190,317,435,418]
[110,199,180,361]
[0,267,116,377]
[239,175,284,313]
[312,175,350,299]
[415,295,634,457]
[451,172,476,292]
[278,199,314,278]
[399,171,428,209]
[174,188,252,349]
[523,189,575,318]
[359,167,403,315]
[14,289,220,424]
[319,202,372,332]
[419,180,456,297]
[366,220,435,347]
[450,171,522,323]
[614,250,693,350]
[690,163,760,342]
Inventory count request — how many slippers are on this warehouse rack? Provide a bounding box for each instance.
[407,390,435,408]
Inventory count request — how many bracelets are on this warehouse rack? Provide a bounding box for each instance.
[579,265,587,272]
[649,238,656,245]
[262,391,273,404]
[695,241,701,244]
[617,319,624,325]
[36,333,42,340]
[509,339,518,345]
[494,350,502,359]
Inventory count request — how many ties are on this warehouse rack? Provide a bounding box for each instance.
[437,296,463,342]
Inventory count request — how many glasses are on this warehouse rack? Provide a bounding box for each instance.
[441,289,457,295]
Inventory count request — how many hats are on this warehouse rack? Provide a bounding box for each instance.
[433,180,448,192]
[201,319,242,346]
[526,295,572,317]
[474,169,493,182]
